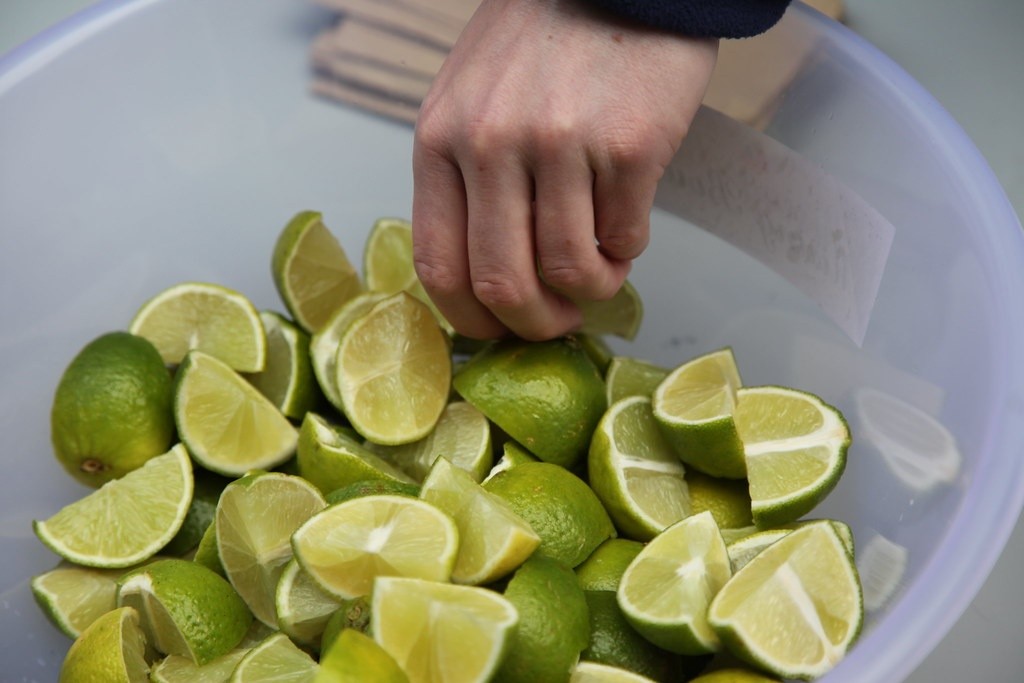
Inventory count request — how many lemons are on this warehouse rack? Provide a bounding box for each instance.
[31,210,865,683]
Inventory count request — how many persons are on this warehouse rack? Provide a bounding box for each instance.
[411,0,793,341]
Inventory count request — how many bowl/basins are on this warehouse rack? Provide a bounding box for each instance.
[0,0,1024,683]
